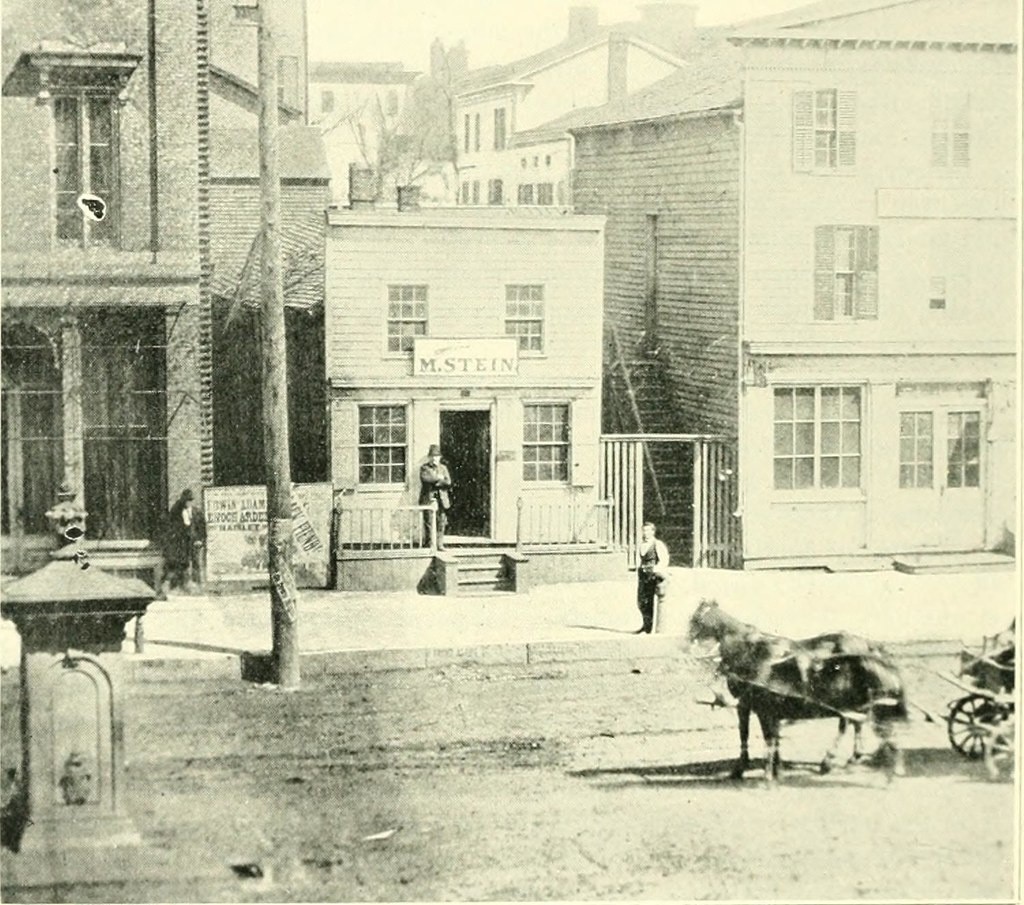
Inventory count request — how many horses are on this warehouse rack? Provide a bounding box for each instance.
[689,596,906,783]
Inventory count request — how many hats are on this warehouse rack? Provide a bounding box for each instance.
[182,489,194,501]
[428,444,442,456]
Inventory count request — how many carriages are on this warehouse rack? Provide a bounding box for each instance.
[685,597,1015,785]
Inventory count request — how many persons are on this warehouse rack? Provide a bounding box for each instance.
[636,522,670,635]
[419,444,451,551]
[173,489,205,590]
